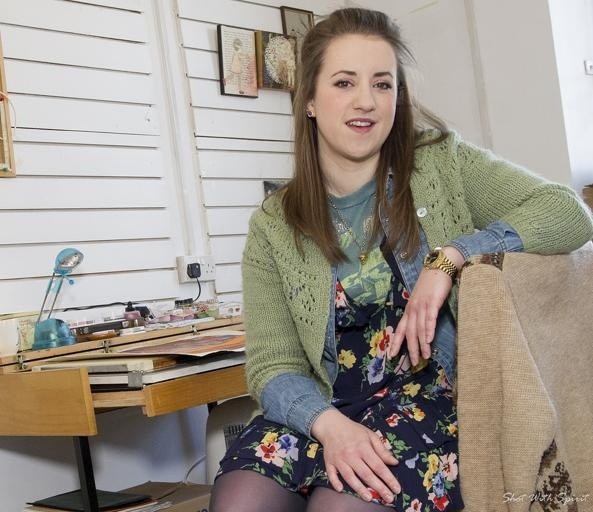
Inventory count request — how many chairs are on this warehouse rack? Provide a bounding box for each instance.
[457,240,593,512]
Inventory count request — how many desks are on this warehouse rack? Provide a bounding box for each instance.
[0,313,249,512]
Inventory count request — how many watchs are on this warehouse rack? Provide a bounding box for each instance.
[421,248,459,279]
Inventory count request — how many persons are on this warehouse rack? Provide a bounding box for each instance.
[206,6,592,512]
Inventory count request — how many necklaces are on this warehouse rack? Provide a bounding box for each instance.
[326,193,378,264]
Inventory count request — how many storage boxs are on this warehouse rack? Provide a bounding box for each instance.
[24,480,210,512]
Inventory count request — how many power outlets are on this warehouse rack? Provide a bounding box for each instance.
[178,254,216,283]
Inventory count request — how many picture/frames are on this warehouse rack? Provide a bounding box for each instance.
[0,29,17,177]
[217,5,315,115]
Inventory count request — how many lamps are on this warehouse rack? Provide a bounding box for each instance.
[31,247,84,350]
